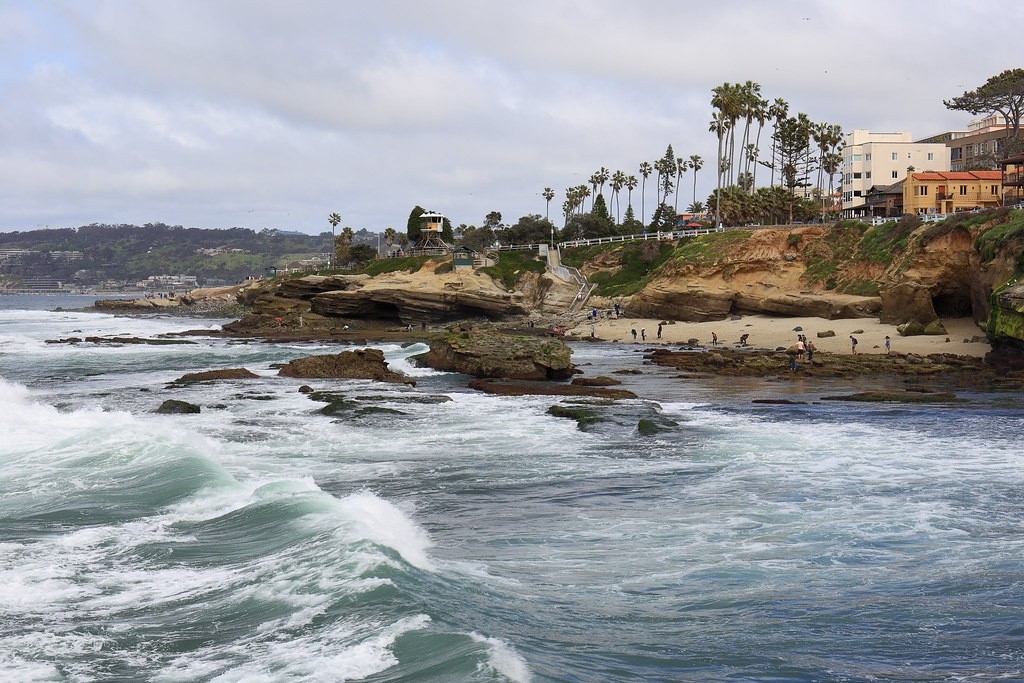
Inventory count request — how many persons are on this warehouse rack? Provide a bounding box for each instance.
[590,325,594,337]
[802,335,806,348]
[658,324,662,338]
[299,315,303,327]
[527,322,530,328]
[548,323,568,336]
[643,230,647,234]
[422,321,426,332]
[407,322,414,333]
[641,328,646,341]
[786,354,799,371]
[739,334,749,344]
[342,324,350,331]
[274,317,282,325]
[798,335,802,341]
[632,329,637,339]
[850,335,857,354]
[807,341,815,360]
[795,339,806,360]
[144,291,176,299]
[885,336,891,351]
[587,303,620,320]
[712,332,718,346]
[530,321,534,328]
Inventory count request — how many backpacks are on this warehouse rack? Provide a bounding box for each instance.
[854,339,857,344]
